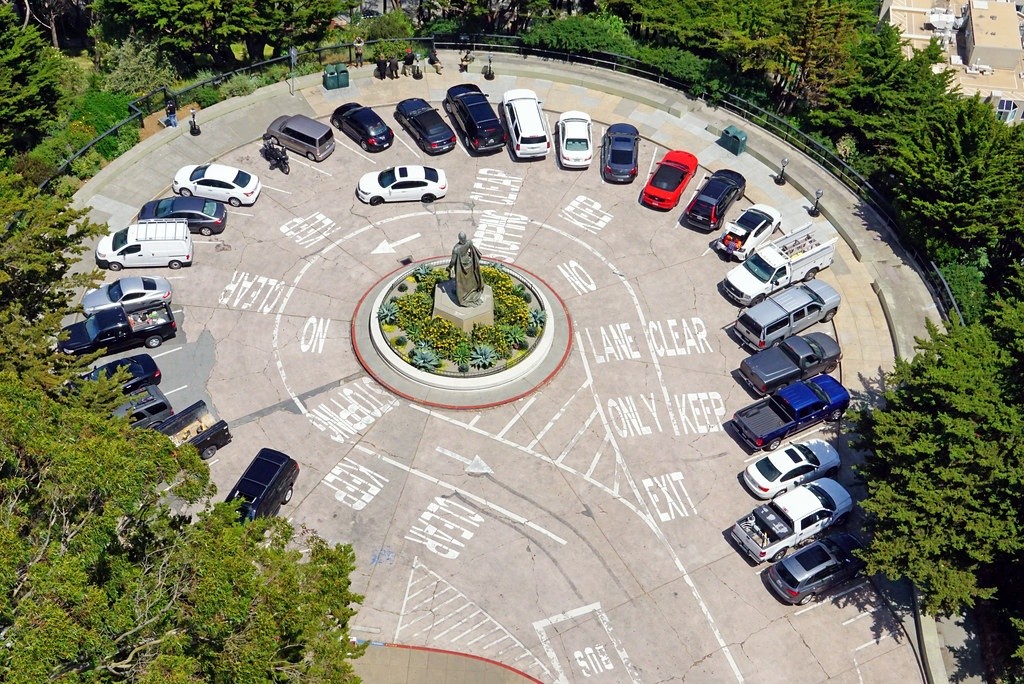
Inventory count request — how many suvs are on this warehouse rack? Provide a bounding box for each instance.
[265,113,337,163]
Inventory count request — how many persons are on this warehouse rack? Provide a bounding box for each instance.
[388,56,400,79]
[377,55,387,80]
[165,97,178,127]
[353,37,364,67]
[429,49,444,75]
[458,50,475,73]
[446,232,482,306]
[403,48,414,76]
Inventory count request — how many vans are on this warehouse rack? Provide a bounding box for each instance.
[112,383,174,432]
[221,447,301,542]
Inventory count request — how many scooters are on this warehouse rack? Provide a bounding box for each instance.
[263,133,291,175]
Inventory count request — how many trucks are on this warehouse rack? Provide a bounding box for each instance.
[721,221,836,306]
[95,218,194,272]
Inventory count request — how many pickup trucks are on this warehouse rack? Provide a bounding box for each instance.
[732,278,842,355]
[154,399,233,460]
[737,332,844,397]
[730,477,854,565]
[57,298,178,359]
[728,373,851,452]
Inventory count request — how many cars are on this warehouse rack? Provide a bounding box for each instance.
[444,83,509,153]
[716,203,784,263]
[742,438,843,501]
[392,97,457,156]
[60,353,162,404]
[766,530,868,606]
[172,162,262,208]
[355,164,449,206]
[556,110,595,170]
[137,195,228,236]
[329,102,396,154]
[684,168,747,232]
[501,88,552,160]
[641,148,699,210]
[80,276,173,319]
[602,123,641,184]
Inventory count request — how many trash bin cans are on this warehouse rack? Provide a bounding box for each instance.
[322,64,338,90]
[334,63,349,88]
[721,125,748,156]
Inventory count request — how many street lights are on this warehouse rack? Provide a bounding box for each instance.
[484,54,495,80]
[188,107,202,136]
[412,53,424,80]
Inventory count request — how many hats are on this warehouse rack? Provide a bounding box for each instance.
[406,49,411,53]
